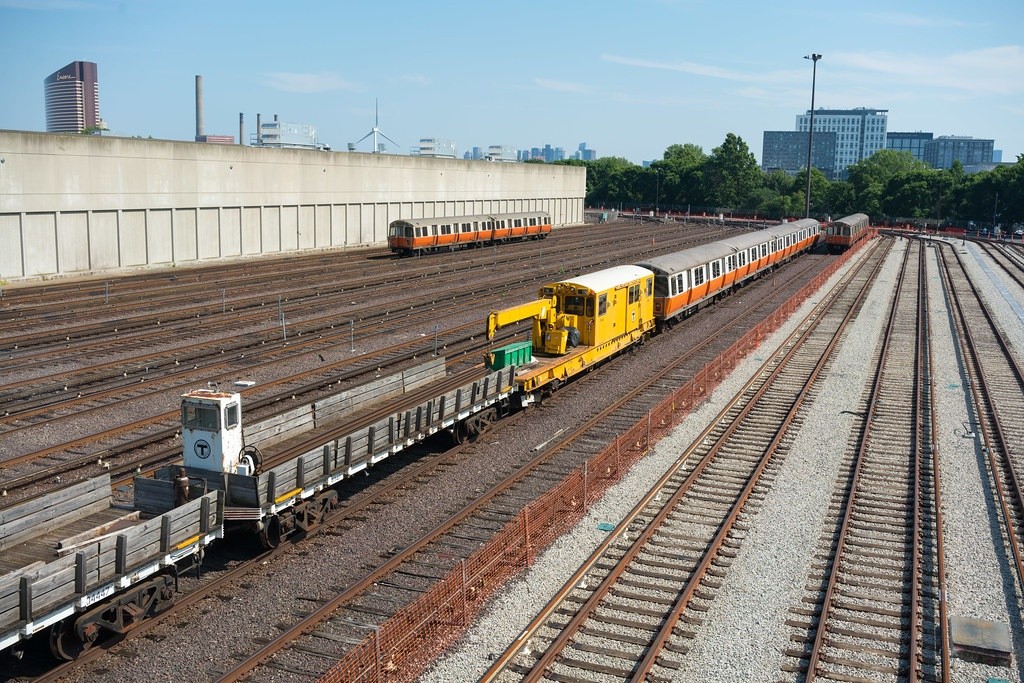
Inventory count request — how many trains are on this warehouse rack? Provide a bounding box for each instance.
[825,213,870,254]
[388,211,551,257]
[0,217,821,662]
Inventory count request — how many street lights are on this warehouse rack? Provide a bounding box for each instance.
[804,54,823,217]
